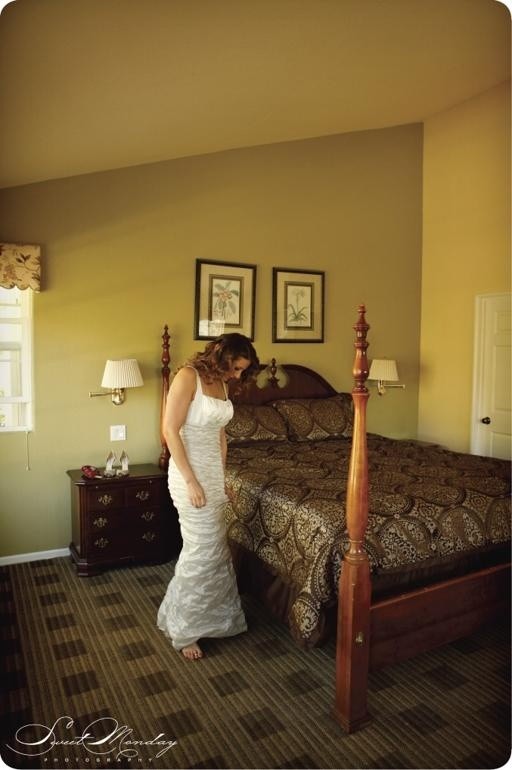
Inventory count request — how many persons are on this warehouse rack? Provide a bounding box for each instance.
[156,332,259,661]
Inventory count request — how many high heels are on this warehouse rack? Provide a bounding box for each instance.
[103,450,116,479]
[116,449,129,478]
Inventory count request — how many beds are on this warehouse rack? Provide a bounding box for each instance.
[157,302,511,734]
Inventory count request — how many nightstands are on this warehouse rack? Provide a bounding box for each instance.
[64,464,175,578]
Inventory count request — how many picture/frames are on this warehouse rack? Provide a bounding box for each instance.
[271,266,326,343]
[193,257,258,344]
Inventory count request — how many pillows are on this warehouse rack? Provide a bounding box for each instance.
[224,402,288,445]
[270,391,355,442]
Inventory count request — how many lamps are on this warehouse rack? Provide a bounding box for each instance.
[89,358,145,407]
[367,358,406,396]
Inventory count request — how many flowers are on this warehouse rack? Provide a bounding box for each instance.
[287,287,310,324]
